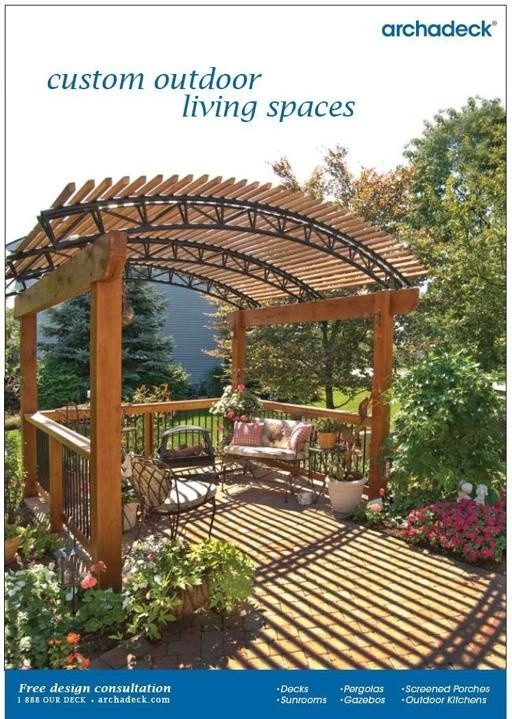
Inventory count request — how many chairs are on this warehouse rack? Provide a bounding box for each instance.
[158,424,216,478]
[121,453,216,544]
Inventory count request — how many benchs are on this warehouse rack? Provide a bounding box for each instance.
[221,418,313,502]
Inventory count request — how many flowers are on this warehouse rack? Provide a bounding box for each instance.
[210,380,261,422]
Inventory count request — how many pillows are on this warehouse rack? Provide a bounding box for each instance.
[232,421,265,446]
[289,423,314,452]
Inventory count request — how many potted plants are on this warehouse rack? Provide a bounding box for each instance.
[4,452,24,564]
[324,431,369,513]
[316,419,337,448]
[127,540,255,641]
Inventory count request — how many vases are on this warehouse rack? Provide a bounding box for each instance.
[223,418,234,428]
